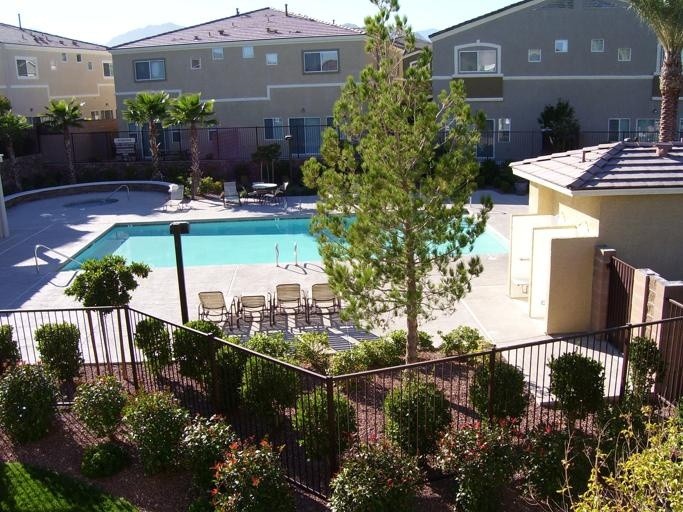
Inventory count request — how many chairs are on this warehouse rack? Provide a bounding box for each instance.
[245,181,290,208]
[161,183,189,213]
[306,283,341,324]
[197,291,236,331]
[234,291,273,327]
[272,283,307,324]
[221,181,242,208]
